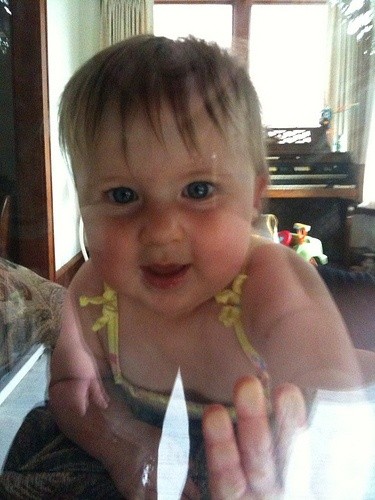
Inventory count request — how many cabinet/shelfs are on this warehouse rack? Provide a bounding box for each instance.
[259,126,360,270]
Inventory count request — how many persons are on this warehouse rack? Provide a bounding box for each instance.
[49,35,375,500]
